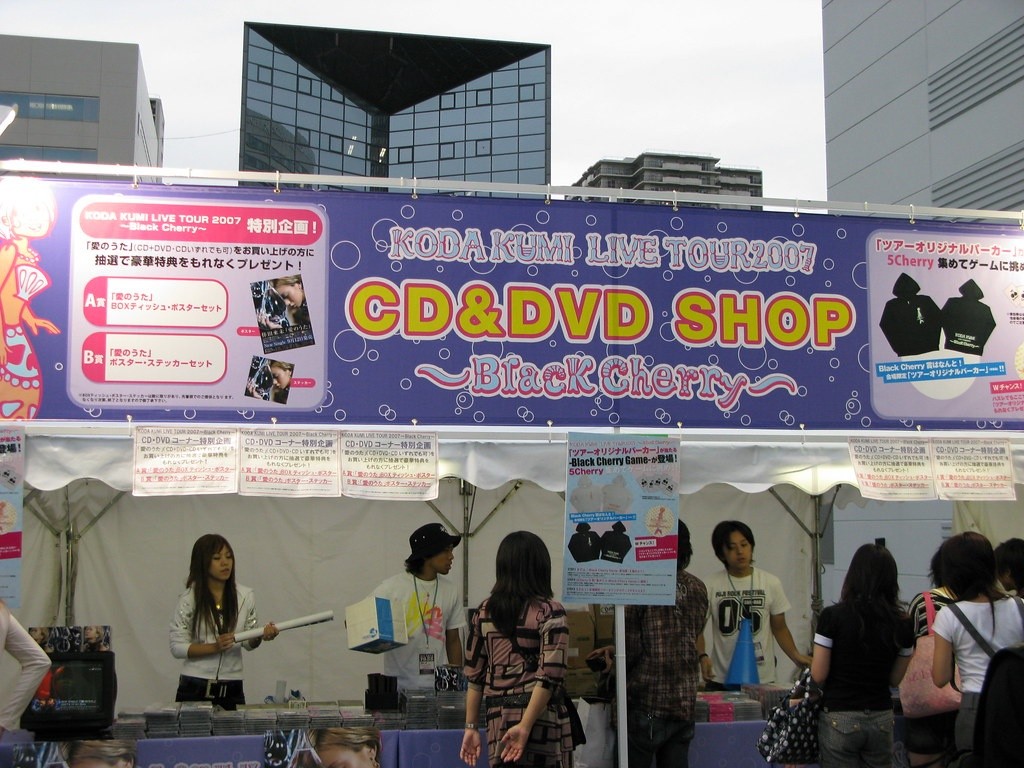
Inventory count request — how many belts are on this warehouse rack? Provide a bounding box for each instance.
[179,675,244,697]
[486,694,564,708]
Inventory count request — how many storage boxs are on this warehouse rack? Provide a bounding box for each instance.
[346,595,408,656]
[564,602,616,666]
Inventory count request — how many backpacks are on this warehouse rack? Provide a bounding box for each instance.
[947,596,1023,768]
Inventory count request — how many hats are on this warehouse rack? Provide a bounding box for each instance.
[405,523,462,563]
[678,519,693,555]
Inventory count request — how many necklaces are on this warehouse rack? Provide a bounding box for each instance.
[414,573,439,651]
[210,595,223,609]
[727,570,754,619]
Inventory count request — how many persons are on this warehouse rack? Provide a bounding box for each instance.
[601,516,709,768]
[169,533,280,711]
[28,626,105,714]
[695,521,812,691]
[459,530,586,768]
[60,739,136,768]
[346,523,468,689]
[810,531,1024,768]
[246,276,311,402]
[1,599,51,734]
[299,728,383,768]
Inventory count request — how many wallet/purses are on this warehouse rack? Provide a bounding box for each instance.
[585,657,607,672]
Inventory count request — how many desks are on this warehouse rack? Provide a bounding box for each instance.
[399,720,774,768]
[0,730,401,768]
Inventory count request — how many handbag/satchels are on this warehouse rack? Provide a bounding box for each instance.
[898,592,962,719]
[756,668,824,764]
[564,697,587,746]
[597,671,616,698]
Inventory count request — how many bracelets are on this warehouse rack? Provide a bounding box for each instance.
[464,724,480,728]
[698,653,709,660]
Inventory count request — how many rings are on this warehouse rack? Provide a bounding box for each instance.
[268,636,271,638]
